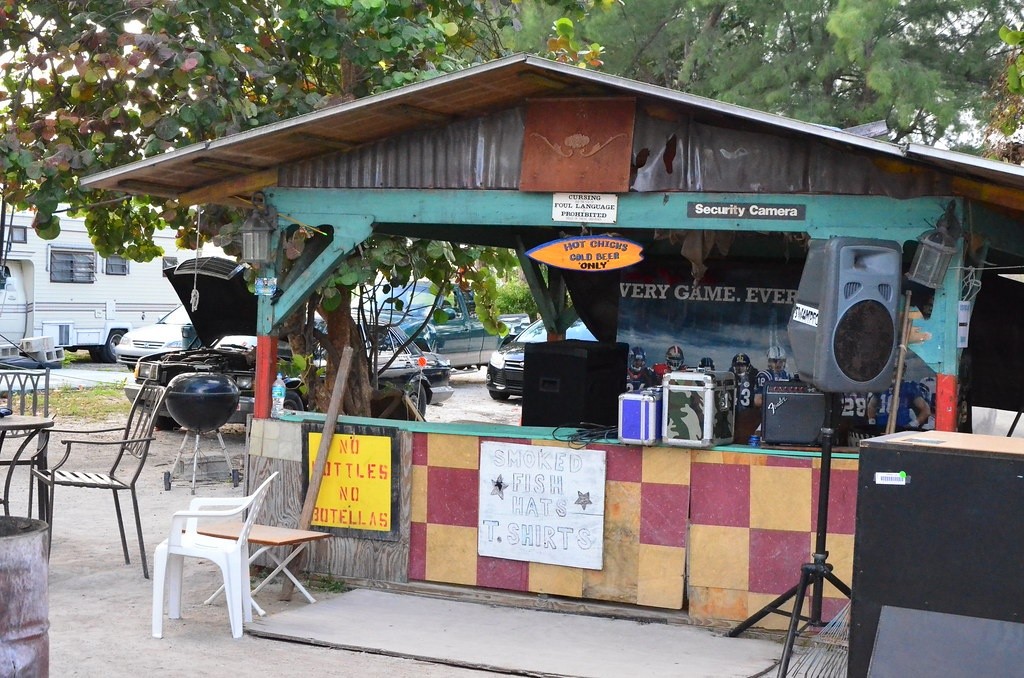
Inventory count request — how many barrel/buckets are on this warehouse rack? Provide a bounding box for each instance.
[0,516,50,678]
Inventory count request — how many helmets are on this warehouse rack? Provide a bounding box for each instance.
[699,357,715,370]
[666,346,685,370]
[629,346,647,373]
[767,346,787,370]
[732,353,751,374]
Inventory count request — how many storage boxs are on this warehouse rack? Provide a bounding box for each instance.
[662,369,738,449]
[619,385,662,445]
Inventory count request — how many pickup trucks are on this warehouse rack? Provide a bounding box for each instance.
[347,280,532,370]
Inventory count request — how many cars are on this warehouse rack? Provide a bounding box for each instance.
[486,317,599,401]
[124,256,454,430]
[114,305,203,370]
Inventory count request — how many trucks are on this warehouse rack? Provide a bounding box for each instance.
[0,212,243,364]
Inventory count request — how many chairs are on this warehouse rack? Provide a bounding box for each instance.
[152,471,279,640]
[0,365,49,520]
[28,378,173,579]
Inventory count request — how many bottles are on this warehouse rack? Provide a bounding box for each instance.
[271,372,286,417]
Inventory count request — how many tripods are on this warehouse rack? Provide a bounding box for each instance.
[726,392,852,678]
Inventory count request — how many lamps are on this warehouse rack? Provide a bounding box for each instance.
[238,206,276,263]
[905,218,957,289]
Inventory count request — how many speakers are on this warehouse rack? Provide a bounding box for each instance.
[787,236,903,394]
[521,339,630,430]
[762,380,843,446]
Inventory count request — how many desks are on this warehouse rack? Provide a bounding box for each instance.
[182,521,333,616]
[0,415,54,516]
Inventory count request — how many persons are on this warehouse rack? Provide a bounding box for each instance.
[628,341,930,448]
